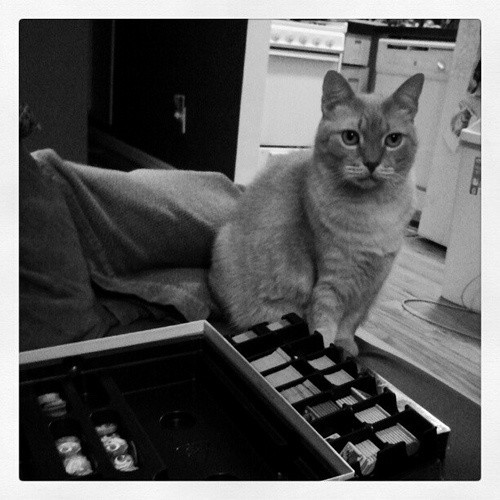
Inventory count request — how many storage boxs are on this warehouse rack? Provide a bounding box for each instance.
[19,319,355,481]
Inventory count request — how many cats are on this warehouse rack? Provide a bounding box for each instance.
[209,71,426,359]
